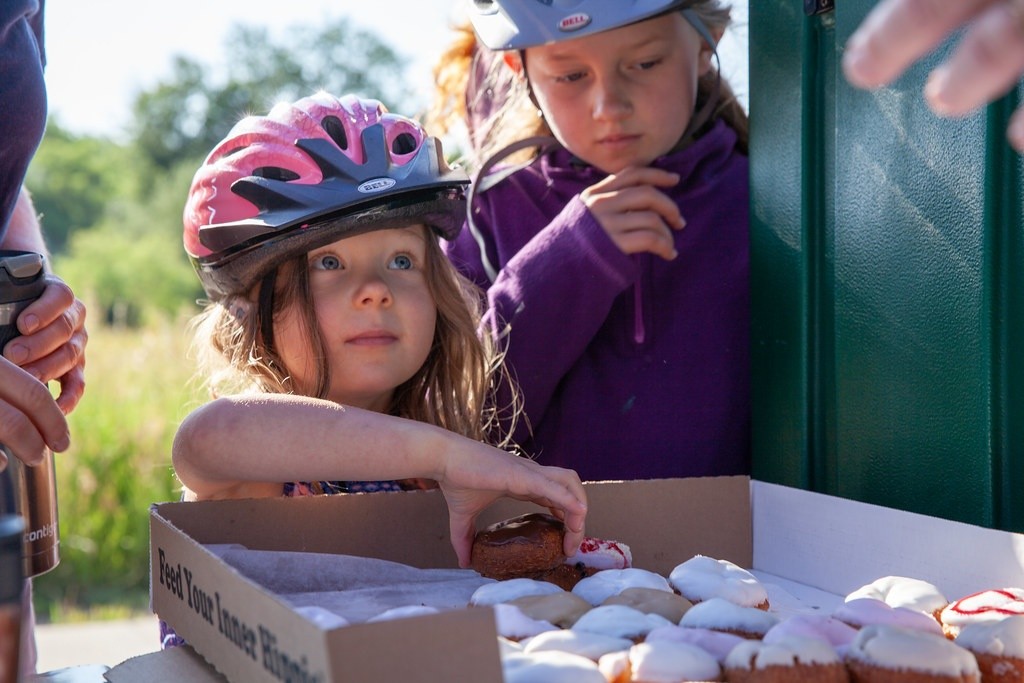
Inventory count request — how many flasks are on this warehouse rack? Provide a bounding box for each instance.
[1,249,60,578]
[1,514,41,680]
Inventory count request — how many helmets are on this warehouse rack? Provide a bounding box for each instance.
[182,94,468,295]
[467,0,704,51]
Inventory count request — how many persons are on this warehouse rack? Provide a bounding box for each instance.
[842,0,1024,155]
[0,0,89,683]
[434,0,749,479]
[170,92,587,568]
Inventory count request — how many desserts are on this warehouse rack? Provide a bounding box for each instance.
[287,511,1024,683]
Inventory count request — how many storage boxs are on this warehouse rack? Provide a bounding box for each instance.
[150,473,1024,683]
